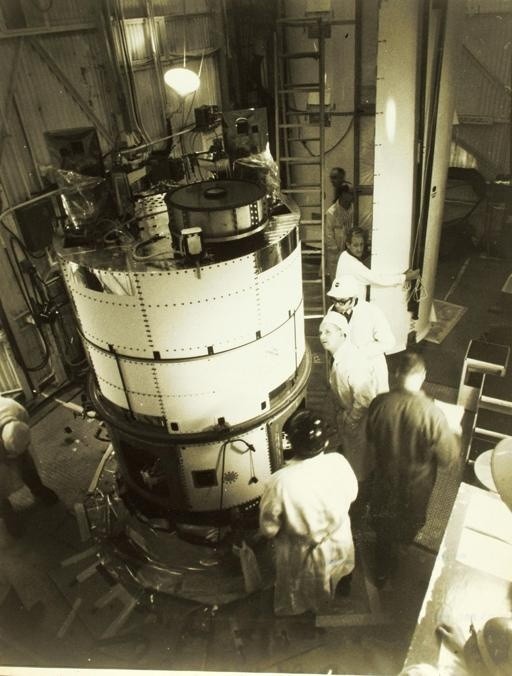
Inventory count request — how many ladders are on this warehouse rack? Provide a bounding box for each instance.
[272,14,326,324]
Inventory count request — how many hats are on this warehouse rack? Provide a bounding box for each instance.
[320,309,350,336]
[325,276,362,298]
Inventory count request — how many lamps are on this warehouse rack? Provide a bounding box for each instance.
[164,67,200,121]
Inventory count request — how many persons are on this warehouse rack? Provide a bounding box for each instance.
[335,226,421,301]
[255,409,360,638]
[364,349,463,588]
[325,274,402,395]
[321,182,356,277]
[0,395,59,538]
[329,166,352,202]
[318,310,379,516]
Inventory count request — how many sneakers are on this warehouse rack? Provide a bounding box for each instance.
[373,556,398,586]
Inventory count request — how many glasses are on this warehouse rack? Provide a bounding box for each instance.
[331,298,350,305]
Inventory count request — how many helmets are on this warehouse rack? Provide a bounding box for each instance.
[287,409,327,459]
